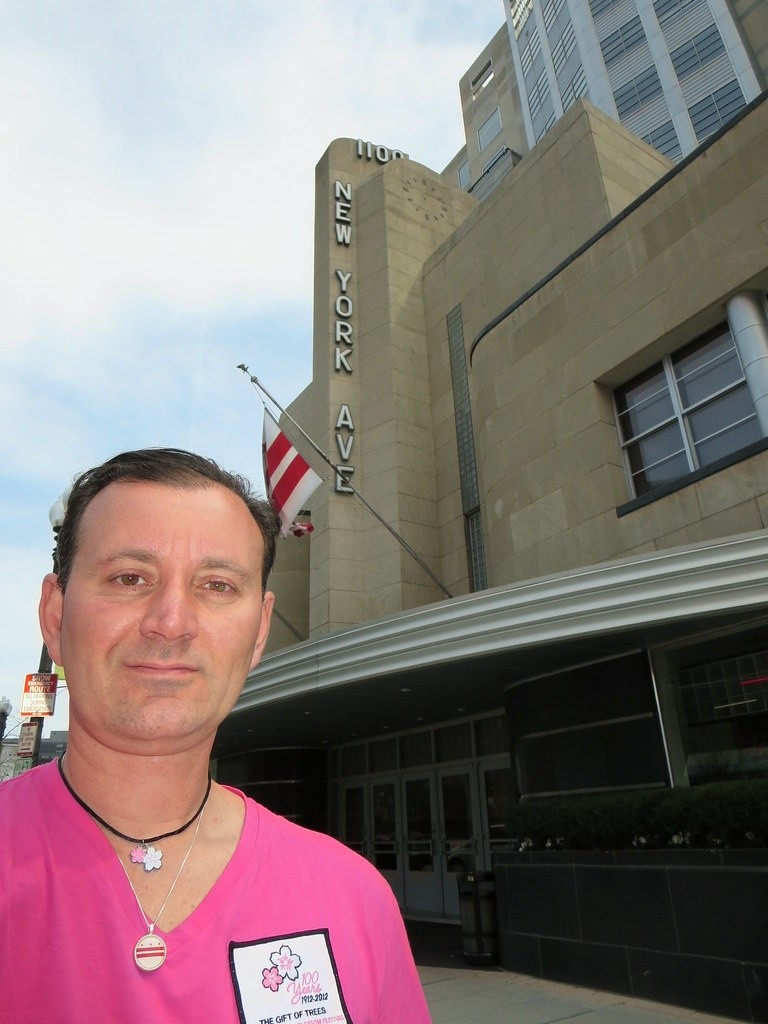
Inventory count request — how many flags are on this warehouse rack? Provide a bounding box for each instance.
[262,407,324,539]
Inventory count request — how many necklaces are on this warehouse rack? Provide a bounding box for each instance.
[57,749,211,872]
[115,804,206,974]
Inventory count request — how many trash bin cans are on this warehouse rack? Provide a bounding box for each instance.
[456,869,502,969]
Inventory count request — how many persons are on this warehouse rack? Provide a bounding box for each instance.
[0,446,432,1024]
